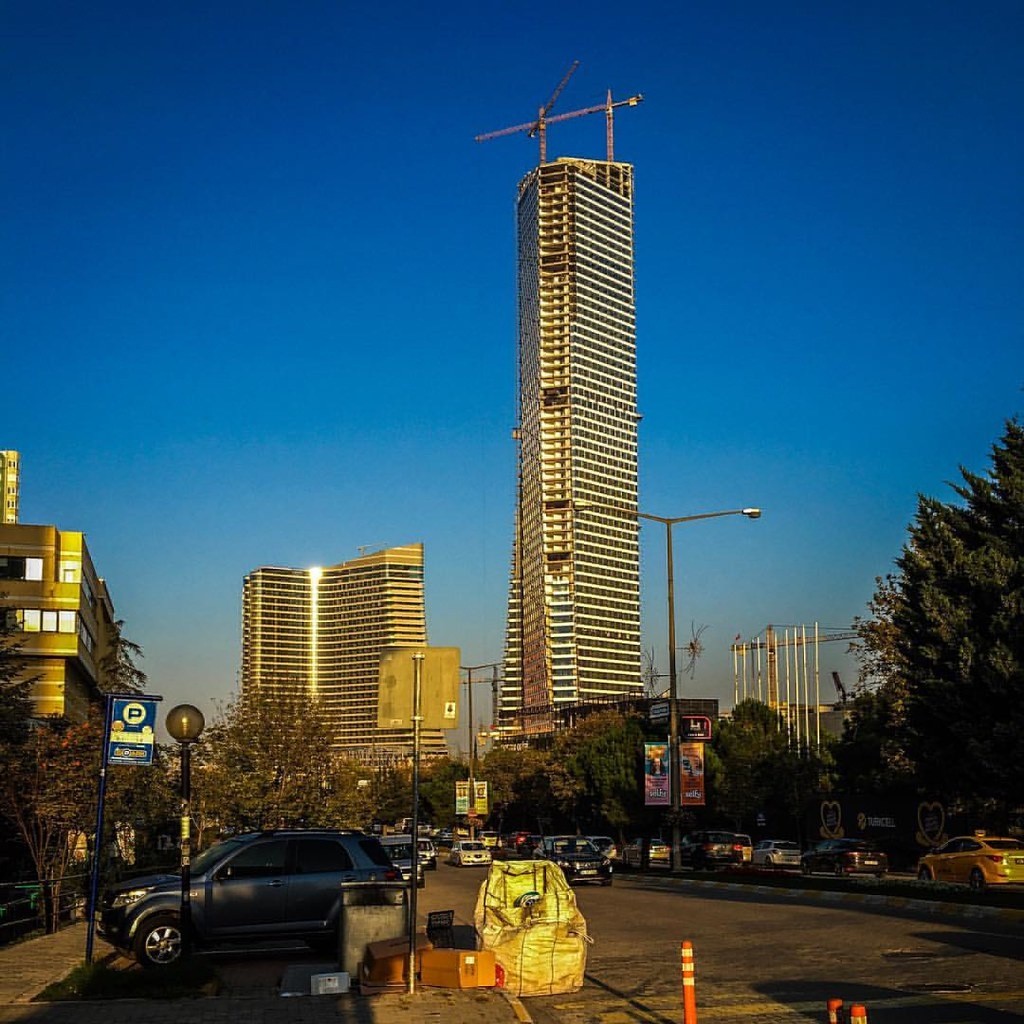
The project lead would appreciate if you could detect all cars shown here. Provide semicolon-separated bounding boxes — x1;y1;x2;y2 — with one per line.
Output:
507;830;540;858
800;838;889;877
533;836;613;887
752;838;801;869
918;830;1024;890
475;830;503;850
451;840;493;867
399;816;470;844
418;838;440;871
585;836;617;860
621;838;671;865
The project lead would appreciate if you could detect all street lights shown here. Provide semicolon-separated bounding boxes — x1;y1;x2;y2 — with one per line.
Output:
165;702;207;999
568;499;762;873
459;658;519;840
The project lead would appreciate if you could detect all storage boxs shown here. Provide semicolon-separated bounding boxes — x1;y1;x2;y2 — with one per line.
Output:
364;911;497;988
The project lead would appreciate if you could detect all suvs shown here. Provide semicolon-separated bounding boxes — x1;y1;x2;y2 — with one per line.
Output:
95;830;408;982
680;830;744;874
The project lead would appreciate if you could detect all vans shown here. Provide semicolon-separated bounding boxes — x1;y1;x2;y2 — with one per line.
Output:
379;835;429;889
733;833;754;870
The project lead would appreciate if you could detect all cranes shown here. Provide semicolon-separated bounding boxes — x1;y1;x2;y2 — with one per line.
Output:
467;52;649;167
727;622;866;711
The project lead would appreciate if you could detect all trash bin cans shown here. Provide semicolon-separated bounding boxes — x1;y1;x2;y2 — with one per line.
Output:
340;882;411;979
